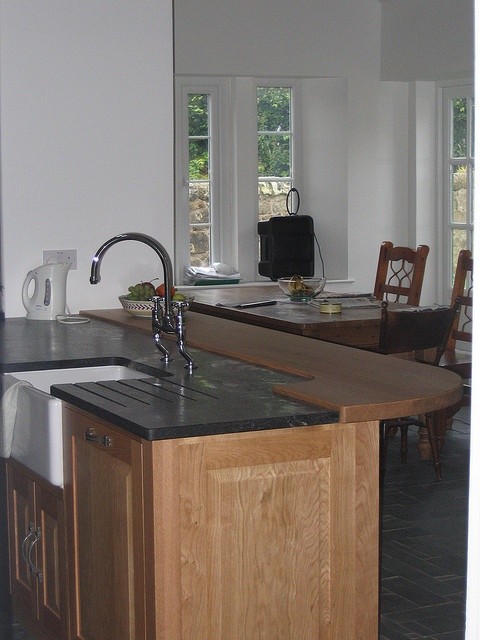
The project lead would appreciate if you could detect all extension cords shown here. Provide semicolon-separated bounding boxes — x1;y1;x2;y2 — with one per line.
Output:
22;260;73;321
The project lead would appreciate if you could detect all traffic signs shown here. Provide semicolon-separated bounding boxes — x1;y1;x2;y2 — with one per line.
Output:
43;249;77;270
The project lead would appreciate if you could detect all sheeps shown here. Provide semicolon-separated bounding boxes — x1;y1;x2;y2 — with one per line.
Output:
89;231;175;334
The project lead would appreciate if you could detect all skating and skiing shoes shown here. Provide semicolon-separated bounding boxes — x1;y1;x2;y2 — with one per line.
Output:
127;284;154;301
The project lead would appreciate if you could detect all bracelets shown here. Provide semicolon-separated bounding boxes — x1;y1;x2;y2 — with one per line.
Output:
278;277;326;302
118;296;195;318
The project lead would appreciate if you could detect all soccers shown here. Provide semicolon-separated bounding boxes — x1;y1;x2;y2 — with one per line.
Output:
156;282;175;297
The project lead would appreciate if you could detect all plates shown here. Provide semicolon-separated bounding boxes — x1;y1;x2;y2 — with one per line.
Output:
64;400;379;640
0;455;66;639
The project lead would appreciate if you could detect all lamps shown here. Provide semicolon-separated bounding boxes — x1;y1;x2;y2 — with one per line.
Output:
373;242;429;306
437;250;472;454
377;301;462;481
